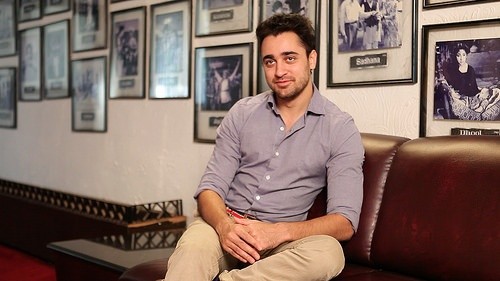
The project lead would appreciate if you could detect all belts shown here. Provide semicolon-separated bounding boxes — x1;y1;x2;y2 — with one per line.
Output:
225;204;262;221
345;21;356;24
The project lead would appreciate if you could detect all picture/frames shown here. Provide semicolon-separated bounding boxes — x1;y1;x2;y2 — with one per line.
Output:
0;0;322;144
422;0;484;8
419;18;500;137
325;0;418;88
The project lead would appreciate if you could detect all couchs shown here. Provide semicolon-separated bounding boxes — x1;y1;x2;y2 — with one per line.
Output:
118;133;500;281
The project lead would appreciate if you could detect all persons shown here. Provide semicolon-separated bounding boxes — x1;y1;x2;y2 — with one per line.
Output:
206;57;243;111
340;0;399;49
448;45;493;112
162;14;365;281
271;0;293;14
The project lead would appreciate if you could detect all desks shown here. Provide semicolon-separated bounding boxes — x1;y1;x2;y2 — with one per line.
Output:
47;228;184;281
